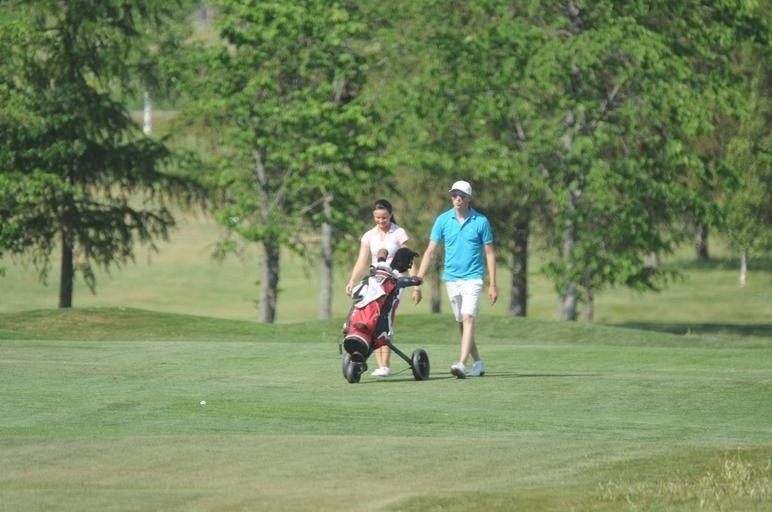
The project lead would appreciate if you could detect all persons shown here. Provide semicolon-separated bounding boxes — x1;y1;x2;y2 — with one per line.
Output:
345;199;416;379
413;180;497;378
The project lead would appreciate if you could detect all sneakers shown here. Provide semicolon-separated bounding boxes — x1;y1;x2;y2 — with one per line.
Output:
468;361;486;376
451;363;466;379
372;368;390;377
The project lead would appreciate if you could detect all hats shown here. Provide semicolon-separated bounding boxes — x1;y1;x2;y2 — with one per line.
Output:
448;180;473;197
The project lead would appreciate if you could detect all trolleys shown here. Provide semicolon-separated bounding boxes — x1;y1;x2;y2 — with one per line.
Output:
341;274;431;385
